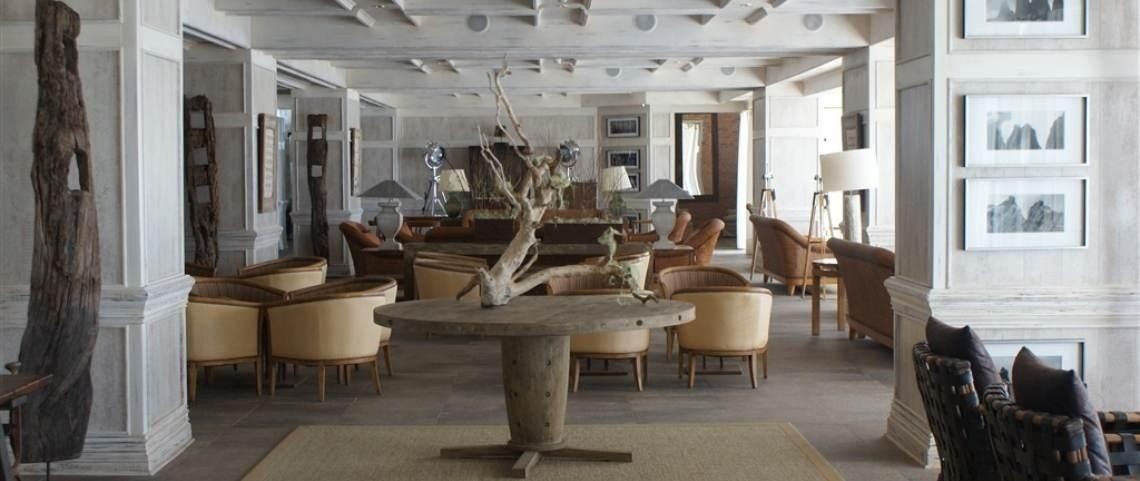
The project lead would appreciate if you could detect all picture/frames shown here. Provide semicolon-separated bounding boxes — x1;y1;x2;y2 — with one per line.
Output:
607;150;640;170
963;178;1087;251
607;117;640;138
961;1;1088;41
351;127;361;196
258;113;278;213
962;95;1090;168
628;172;640;192
841;113;863;150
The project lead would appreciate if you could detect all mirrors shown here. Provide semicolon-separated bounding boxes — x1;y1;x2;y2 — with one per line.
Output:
675;112;719;204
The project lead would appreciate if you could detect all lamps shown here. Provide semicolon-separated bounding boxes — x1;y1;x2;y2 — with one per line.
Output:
599;166;632;218
635;179;695;250
820;147;881;246
438;169;471;225
357;180;423;251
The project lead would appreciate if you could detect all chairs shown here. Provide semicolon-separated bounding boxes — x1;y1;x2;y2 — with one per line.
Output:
911;313;1140;481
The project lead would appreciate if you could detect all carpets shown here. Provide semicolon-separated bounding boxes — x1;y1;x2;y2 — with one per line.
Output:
239;421;841;481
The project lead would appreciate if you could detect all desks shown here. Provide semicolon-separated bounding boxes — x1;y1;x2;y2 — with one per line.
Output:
372;294;696;480
0;372;55;481
